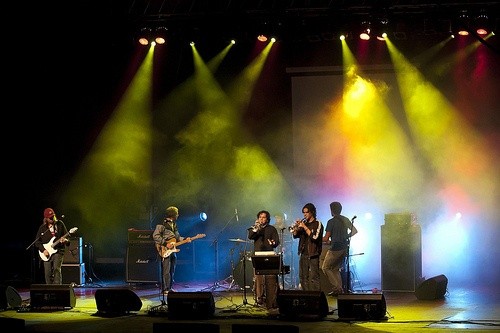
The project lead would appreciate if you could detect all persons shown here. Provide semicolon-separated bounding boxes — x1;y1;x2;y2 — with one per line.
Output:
36;208;70;284
293;203;324;290
153;206;191;293
272;211;292;265
248;210;280;305
322;202;358;296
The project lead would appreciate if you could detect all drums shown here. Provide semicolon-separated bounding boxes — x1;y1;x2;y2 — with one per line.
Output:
233;257;255;286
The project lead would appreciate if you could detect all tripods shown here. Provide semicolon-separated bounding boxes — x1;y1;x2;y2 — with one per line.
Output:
142;223;169;313
200;213;235;292
233;226;264;311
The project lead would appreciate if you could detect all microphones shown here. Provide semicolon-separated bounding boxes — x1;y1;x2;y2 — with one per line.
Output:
235;208;239;222
352;215;357;220
164;219;173;222
56;215;65;221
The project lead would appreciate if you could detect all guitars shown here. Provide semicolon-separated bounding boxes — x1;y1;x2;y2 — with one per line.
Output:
155;234;205;259
38;227;77;261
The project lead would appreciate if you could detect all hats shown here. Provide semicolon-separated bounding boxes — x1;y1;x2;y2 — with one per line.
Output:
44;208;55;218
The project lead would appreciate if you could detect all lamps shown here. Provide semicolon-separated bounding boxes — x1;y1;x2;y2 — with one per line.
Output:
139;8;491;45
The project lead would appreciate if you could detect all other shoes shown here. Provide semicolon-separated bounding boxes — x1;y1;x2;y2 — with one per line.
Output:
255;302;264;307
160;289;175;295
328;291;339;295
266;307;279;311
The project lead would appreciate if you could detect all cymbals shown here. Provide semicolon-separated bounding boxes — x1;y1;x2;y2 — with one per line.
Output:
229;239;245;242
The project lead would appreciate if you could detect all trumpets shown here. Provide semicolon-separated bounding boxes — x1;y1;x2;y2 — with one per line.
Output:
288;216;307;233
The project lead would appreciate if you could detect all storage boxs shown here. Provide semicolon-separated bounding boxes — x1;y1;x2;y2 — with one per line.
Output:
126;244;163;286
61;262;85;285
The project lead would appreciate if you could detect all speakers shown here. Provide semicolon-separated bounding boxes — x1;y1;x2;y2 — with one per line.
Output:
0;285;22;307
167;291;215;317
337;293;386;320
125;245;161;283
414;274;448;299
62;237;83;264
94;288;141;314
30;284;76;310
277;289;328;319
